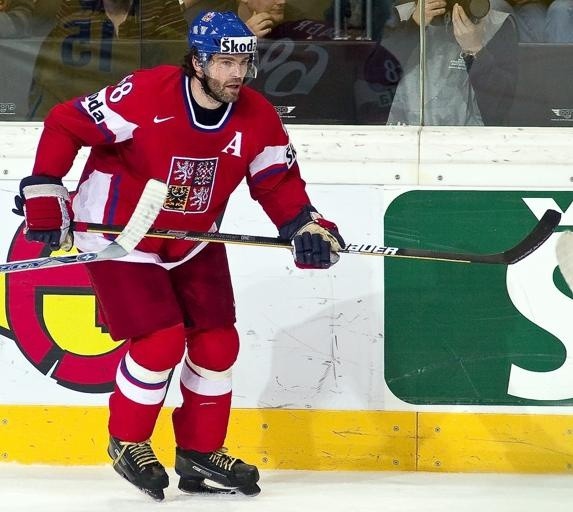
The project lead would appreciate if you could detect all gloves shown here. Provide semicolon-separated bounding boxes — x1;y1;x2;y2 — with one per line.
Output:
278;204;346;270
12;174;76;253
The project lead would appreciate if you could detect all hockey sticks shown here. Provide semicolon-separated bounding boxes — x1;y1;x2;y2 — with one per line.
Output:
0;178;167;273
69;209;561;264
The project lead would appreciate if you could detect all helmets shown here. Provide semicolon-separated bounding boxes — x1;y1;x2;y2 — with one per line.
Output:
188;8;260;80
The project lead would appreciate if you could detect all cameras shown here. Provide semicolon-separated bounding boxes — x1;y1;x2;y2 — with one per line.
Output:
428;0;490;27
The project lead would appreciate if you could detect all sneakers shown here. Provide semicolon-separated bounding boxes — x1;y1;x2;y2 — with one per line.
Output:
175;446;259;487
108;440;170;490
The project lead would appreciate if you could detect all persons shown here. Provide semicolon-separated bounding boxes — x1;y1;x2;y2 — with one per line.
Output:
1;1;573;128
17;7;346;492
